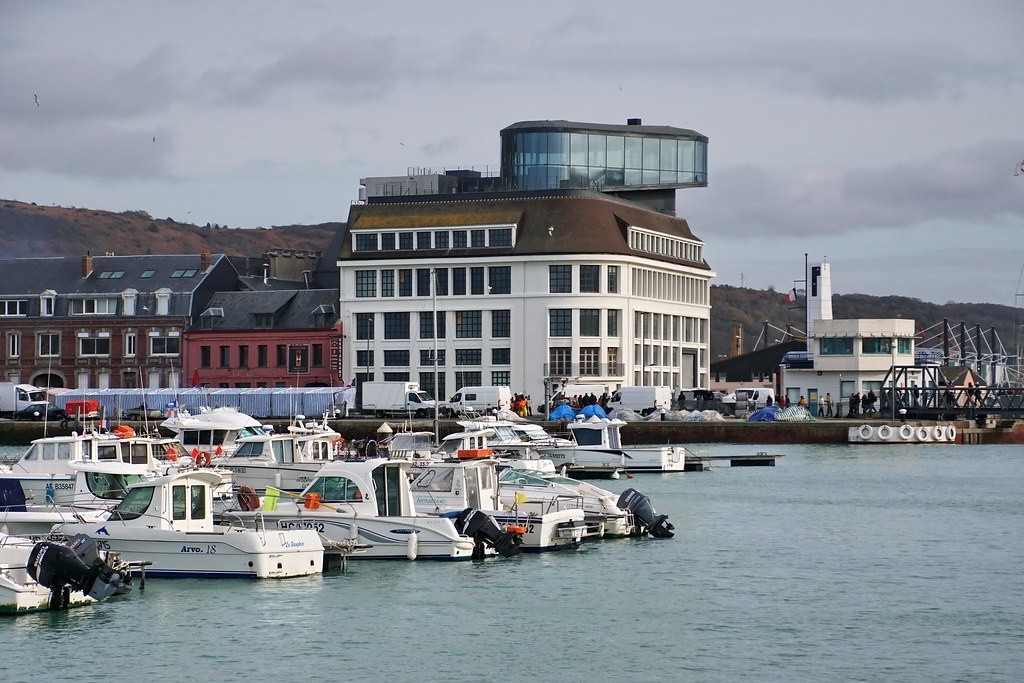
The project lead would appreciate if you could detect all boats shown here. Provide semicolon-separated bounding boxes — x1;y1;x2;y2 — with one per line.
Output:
0;321;688;580
0;504;153;617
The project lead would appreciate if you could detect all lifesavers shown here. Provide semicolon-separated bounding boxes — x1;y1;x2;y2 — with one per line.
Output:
332;437;346;451
237;484;260;511
899;425;914;439
946;426;956;440
931;426;944;440
196;452;211;466
166;448;178;461
507;526;525;533
916;427;930;441
859;425;873;440
878;425;892;439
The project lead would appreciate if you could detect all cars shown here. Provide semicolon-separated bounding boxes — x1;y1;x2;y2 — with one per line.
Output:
12;403;66;422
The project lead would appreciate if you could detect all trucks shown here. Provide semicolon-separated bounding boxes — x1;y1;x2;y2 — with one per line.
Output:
0;380;48;420
362;381;436;419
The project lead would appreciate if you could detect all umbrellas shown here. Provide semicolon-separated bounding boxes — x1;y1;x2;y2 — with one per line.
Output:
192;367;200;388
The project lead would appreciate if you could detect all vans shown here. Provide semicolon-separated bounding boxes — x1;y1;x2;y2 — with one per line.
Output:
444;385;512;419
603;386;672;421
722;387;776;414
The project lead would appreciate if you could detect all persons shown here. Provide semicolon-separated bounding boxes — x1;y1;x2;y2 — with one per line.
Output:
766;394;791;409
819;393;834;418
797;395;807;408
849;384;928;415
944;380;985;407
678;391;685;411
510;393;533;417
573;392;609;411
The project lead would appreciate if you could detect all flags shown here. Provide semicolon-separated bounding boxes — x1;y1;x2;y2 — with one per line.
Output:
215;445;222;455
98;420;110;429
786;288;797;304
191;448;199;459
263;486;279;511
